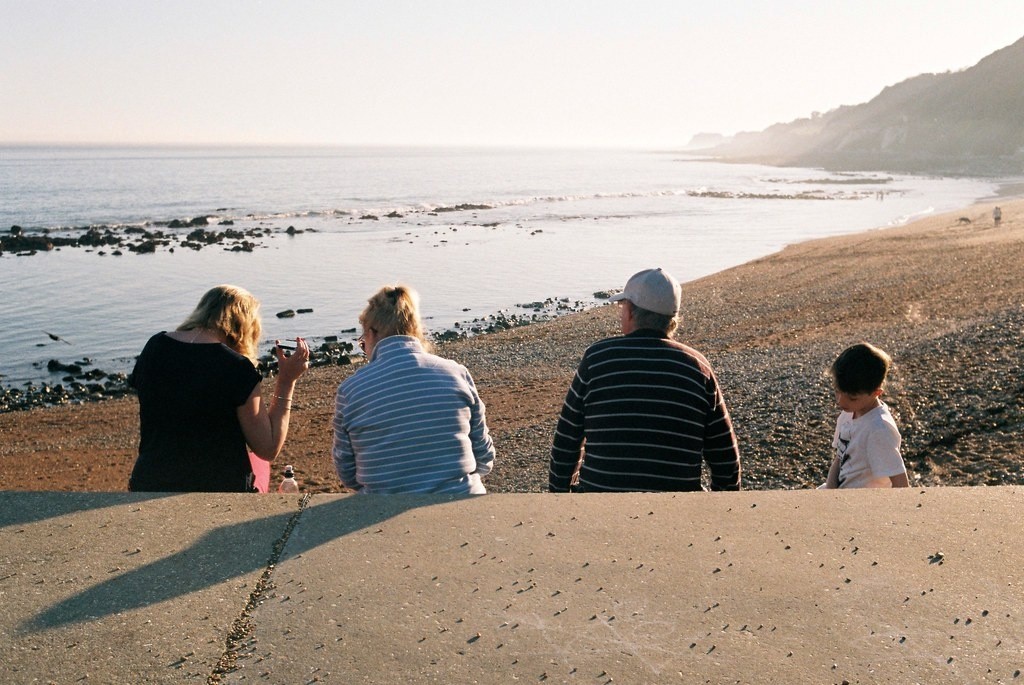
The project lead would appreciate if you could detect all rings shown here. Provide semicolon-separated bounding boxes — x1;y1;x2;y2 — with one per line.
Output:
303;357;309;362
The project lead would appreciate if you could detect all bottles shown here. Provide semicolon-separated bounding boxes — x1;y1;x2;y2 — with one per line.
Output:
279;465;299;493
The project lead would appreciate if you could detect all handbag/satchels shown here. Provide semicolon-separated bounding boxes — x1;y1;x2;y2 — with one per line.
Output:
248;452;270;493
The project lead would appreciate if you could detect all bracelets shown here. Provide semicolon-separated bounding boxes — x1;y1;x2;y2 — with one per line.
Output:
270;393;292;401
270;402;291;411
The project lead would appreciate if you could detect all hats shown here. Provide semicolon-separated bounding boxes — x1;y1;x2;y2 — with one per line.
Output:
609;268;682;315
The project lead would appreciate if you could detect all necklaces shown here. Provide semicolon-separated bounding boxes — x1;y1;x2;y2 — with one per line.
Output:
190;326;217;343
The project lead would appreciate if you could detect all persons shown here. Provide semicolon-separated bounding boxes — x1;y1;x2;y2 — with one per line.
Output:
331;284;496;494
549;267;741;493
816;342;909;488
992;207;1003;227
128;284;310;494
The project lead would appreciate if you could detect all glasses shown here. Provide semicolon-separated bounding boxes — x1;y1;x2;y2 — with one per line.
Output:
357;327;377;352
617;300;632;309
